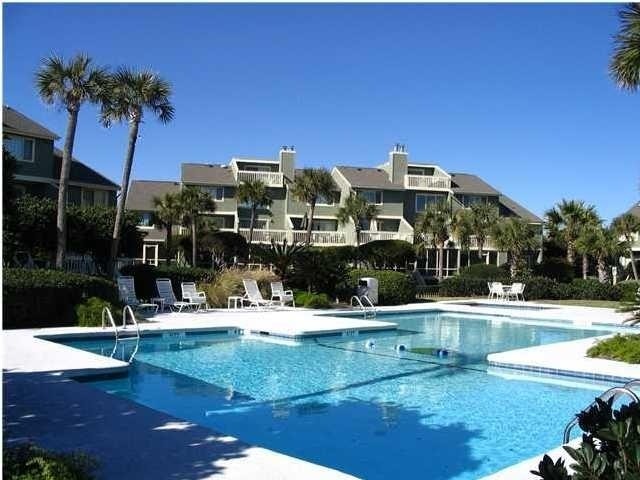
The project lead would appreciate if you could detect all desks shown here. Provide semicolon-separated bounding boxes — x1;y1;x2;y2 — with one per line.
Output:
150;298;165;312
228;296;243;309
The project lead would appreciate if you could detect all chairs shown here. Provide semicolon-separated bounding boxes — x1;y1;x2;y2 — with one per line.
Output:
118;276;159;314
270;281;295;307
242;279;280;307
487;281;526;302
155;278;201;314
181;282;207;311
